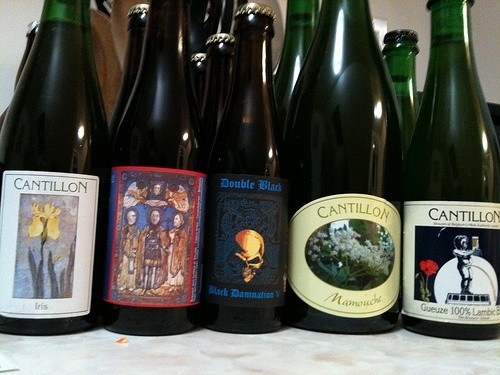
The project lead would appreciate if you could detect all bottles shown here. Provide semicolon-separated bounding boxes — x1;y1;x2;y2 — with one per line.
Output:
402;0;500;340
1;0;109;335
201;4;288;335
272;0;320;143
103;0;209;337
383;31;420;156
15;19;85;104
107;4;151;146
205;34;235;143
189;52;207;108
285;0;405;334
